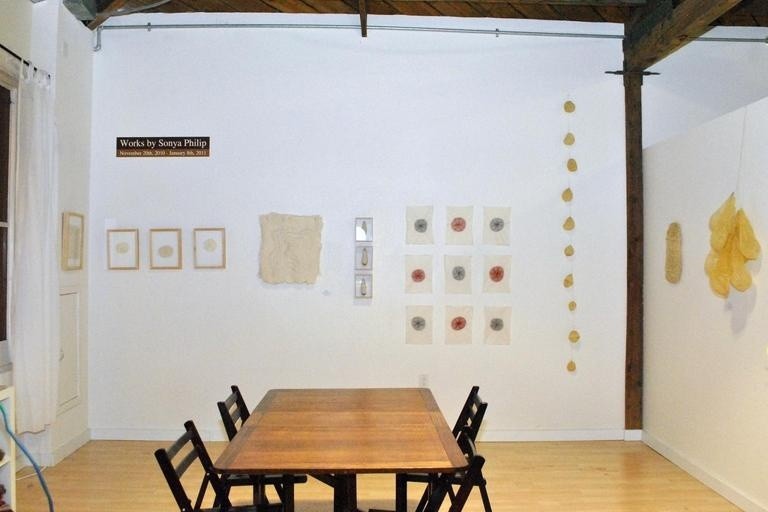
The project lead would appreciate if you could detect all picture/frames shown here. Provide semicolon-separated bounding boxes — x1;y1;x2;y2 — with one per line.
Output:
355;217;374;299
61;210;226;272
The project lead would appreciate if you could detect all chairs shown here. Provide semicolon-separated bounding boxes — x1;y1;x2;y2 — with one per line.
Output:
395;384;488;512
368;427;493;511
153;419;310;511
216;385;297;510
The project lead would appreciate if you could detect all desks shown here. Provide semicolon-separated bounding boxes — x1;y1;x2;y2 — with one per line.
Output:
212;387;468;510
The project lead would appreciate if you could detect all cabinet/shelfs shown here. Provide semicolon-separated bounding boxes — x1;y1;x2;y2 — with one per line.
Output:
0;385;17;512
